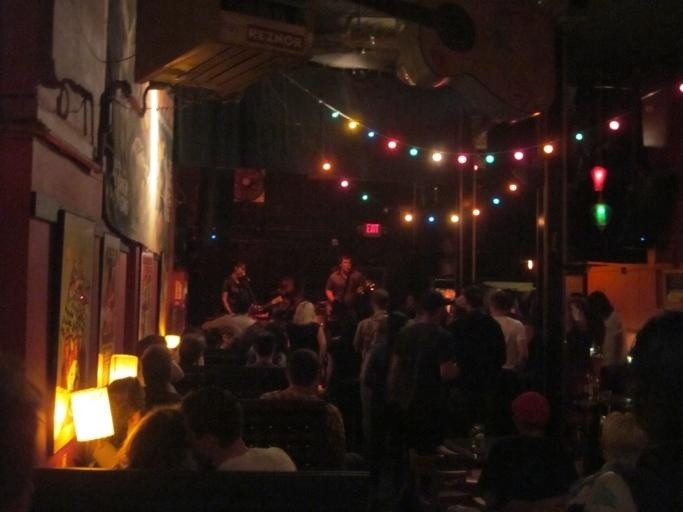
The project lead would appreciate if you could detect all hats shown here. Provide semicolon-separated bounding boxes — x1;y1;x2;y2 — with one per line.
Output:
603;410;646;448
512;391;551;424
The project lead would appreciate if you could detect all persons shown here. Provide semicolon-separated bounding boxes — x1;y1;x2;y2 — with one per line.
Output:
74;254;683;512
0;352;49;512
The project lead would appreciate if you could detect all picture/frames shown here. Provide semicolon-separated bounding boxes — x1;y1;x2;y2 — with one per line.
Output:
46;208;124;460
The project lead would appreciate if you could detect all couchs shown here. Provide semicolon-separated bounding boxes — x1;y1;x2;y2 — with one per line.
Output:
28;364;386;510
